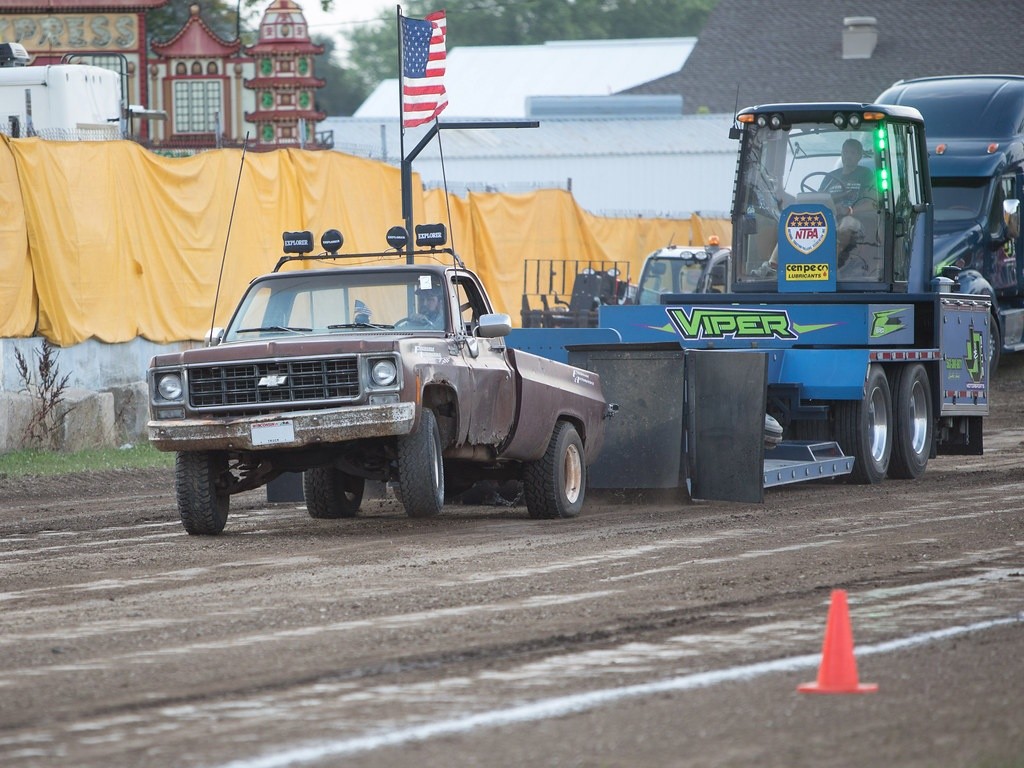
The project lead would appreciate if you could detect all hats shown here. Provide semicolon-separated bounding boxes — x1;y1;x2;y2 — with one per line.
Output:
415;284;441;296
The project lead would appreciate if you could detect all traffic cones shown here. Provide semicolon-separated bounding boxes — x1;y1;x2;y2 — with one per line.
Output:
799;588;879;694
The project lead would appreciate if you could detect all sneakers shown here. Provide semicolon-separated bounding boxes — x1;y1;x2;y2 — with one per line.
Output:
750;263;776;280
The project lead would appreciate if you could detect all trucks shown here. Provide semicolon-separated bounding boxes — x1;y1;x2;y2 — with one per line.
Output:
869;73;1024;380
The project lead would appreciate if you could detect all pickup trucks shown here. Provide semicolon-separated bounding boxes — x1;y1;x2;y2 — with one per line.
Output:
143;223;607;529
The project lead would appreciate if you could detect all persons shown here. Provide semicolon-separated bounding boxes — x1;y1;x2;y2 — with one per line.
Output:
748;139;877;278
402;281;446;330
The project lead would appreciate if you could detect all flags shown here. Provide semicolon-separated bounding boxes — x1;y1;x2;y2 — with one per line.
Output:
399;9;449;129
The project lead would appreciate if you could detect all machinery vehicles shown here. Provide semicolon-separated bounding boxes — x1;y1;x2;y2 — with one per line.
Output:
505;98;988;485
